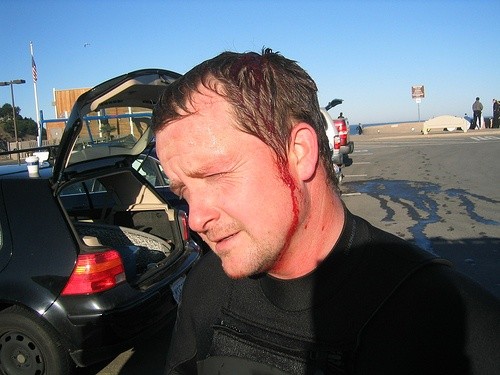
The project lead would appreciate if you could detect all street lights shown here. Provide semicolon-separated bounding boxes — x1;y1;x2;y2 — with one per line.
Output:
0;79;26;165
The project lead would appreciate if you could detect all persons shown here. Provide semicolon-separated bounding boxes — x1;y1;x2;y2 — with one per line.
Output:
493;99;497;126
474;98;482;130
463;112;493;129
337;112;345;119
496;100;499;128
153;47;500;375
358;122;363;135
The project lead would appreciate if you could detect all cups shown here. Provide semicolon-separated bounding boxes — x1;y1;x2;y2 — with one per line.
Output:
25;156;39;177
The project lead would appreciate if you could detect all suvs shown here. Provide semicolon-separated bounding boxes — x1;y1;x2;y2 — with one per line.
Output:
316;106;355;185
0;67;211;375
